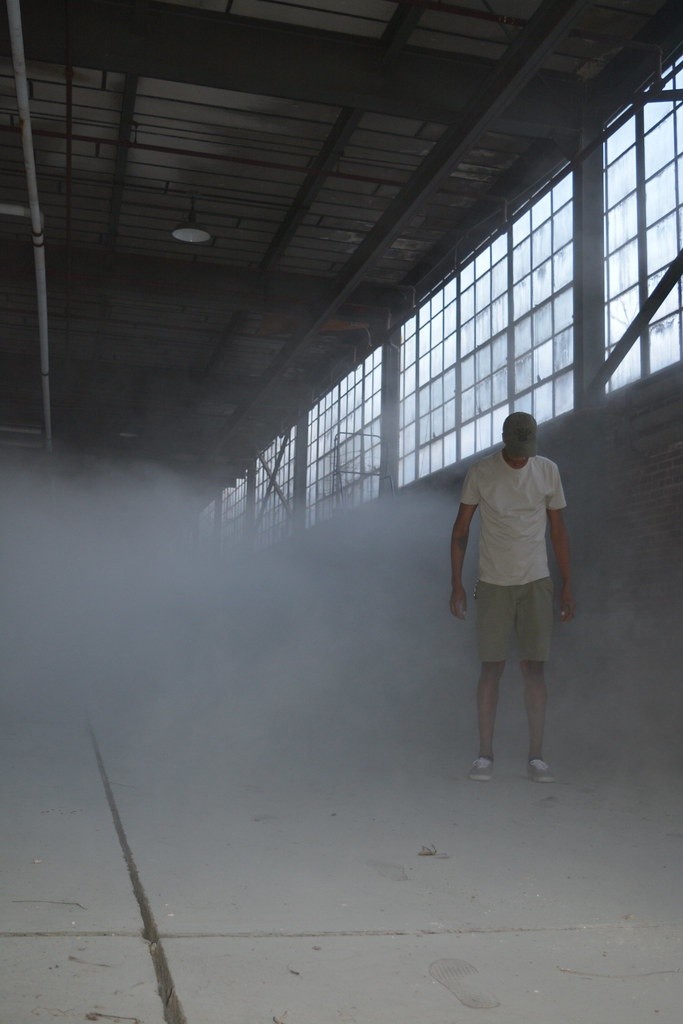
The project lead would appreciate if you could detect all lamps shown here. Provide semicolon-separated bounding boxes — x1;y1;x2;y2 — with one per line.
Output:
169;197;213;243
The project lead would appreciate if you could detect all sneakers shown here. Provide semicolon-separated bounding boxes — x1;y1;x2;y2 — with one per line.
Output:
529;758;557;783
467;755;493;781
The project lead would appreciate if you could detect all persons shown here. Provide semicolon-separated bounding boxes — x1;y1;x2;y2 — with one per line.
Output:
449;412;577;783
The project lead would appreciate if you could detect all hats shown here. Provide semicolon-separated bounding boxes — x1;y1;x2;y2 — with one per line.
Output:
503;410;539;458
